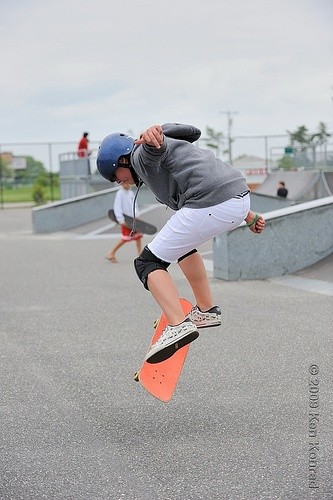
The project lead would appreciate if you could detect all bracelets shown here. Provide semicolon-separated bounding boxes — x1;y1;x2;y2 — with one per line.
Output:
246;214;259;227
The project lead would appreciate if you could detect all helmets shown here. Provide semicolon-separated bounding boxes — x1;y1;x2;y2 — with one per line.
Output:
96;133;135;182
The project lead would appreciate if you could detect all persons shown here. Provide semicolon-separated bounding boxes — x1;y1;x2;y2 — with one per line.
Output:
97;123;265;364
105;183;143;264
277;182;288;198
78;133;89;158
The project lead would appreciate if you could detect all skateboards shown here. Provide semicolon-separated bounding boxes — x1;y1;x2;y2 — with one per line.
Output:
108;209;157;234
133;298;194;402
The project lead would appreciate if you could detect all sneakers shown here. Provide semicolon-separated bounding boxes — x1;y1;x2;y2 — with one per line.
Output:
185;305;222;328
146;316;200;364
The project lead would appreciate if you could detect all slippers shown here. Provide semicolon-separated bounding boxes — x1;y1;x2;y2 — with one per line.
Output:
105;254;117;263
